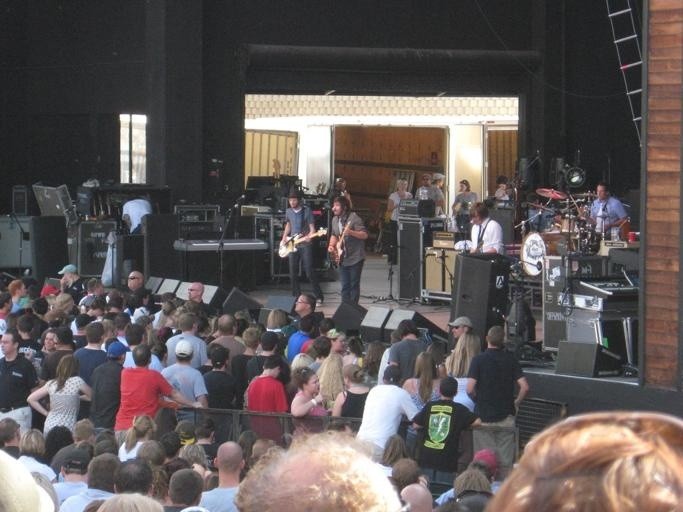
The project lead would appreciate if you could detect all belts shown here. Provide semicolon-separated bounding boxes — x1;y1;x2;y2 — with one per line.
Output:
0;403;28;412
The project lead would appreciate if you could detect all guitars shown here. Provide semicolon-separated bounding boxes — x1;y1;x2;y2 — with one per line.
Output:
278;227;327;258
328;220;352;266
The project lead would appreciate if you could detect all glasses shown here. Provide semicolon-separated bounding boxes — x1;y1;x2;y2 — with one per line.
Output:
455;489;492;501
129;276;138;280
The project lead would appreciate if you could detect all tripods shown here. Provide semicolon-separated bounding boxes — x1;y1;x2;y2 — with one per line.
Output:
372;243;404;306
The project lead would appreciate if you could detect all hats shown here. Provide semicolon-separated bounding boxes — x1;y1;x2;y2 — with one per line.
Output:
212;347;228;362
75;314;96;327
57;264;79;274
448;315;473;328
175;420;195;445
107;342;130;355
488;326;504;344
136;315;155;326
175;340;192;357
64;450;89;469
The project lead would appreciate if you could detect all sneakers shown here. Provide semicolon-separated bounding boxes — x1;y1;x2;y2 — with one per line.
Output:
316;299;322;307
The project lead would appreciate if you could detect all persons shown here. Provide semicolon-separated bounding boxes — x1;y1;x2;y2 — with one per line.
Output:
387;178;414;266
0;265;683;511
121;196;155;264
327;195;369;305
415;174;434;201
468;202;504;256
428;172;448;217
452;179;478;232
588;181;629;241
335;178;352;209
281;192;324;308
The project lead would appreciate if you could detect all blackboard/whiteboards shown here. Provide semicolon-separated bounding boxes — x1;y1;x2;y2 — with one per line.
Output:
78;221;117;278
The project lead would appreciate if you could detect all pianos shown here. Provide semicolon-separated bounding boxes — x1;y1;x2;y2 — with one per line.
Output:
174;237;269;252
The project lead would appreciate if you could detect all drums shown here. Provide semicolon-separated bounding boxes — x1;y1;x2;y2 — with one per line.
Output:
520;230;565;276
562;214;597;232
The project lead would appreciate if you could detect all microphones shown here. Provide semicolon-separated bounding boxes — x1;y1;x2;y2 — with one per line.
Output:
229;205;238;211
233;193;246;205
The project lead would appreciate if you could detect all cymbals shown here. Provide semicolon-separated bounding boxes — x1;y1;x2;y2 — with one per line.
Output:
536;188;567;200
527;201;556;214
572;193;596;198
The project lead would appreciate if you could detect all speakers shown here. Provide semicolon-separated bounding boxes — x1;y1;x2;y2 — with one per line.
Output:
555;341;624;379
331;299;369;337
222;286;264;323
258;295;297;329
145;276;224;310
360;306;393;342
446;251;510;355
28;214;69;279
383;308;449;354
